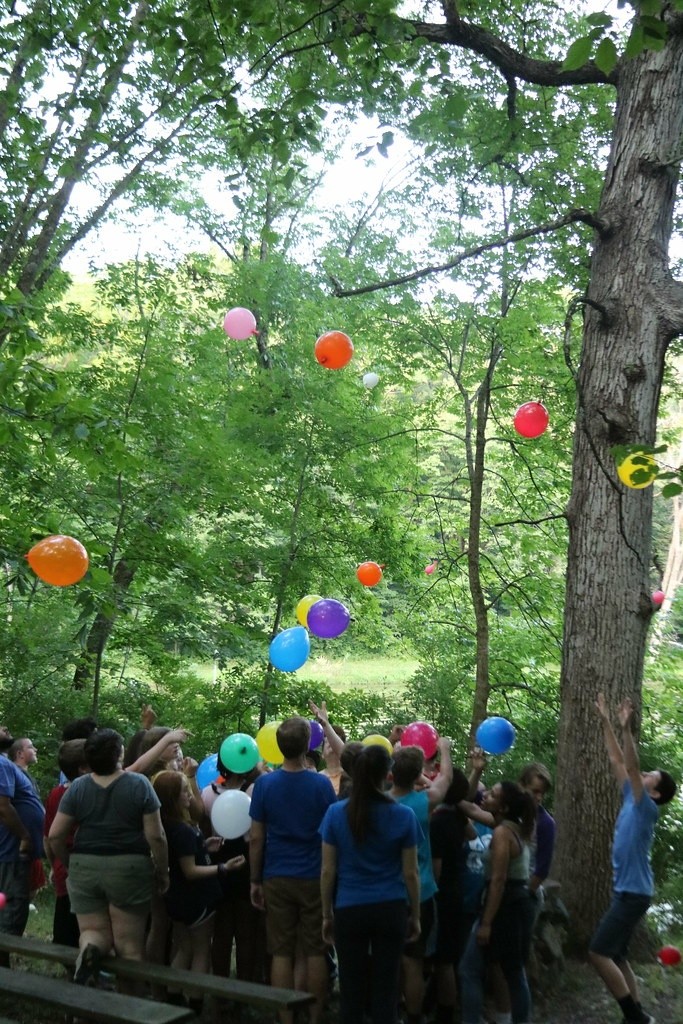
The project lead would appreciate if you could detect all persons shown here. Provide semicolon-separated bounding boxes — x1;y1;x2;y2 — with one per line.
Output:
588;691;677;1024
0;699;556;1024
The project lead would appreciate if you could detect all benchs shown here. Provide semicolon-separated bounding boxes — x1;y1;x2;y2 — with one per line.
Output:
0;932;316;1024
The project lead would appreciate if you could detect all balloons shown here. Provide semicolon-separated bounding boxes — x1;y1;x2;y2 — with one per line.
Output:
401;721;439;761
361;734;393;757
362;373;379;388
296;594;324;627
307;599;351;638
313;329;354;369
210;790;252;841
652;591;665;604
25;535;89;587
617;451;656;489
476;717;516;756
195;721;324;790
268;626;310;672
658;945;682;966
357;561;383;586
224;308;259;340
514;399;549;438
425;566;434;574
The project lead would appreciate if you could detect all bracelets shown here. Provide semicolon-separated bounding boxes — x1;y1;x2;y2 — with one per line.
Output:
218;863;221;875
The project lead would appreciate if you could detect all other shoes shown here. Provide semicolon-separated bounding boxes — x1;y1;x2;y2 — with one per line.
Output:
76;944;102;988
621;1012;655;1024
